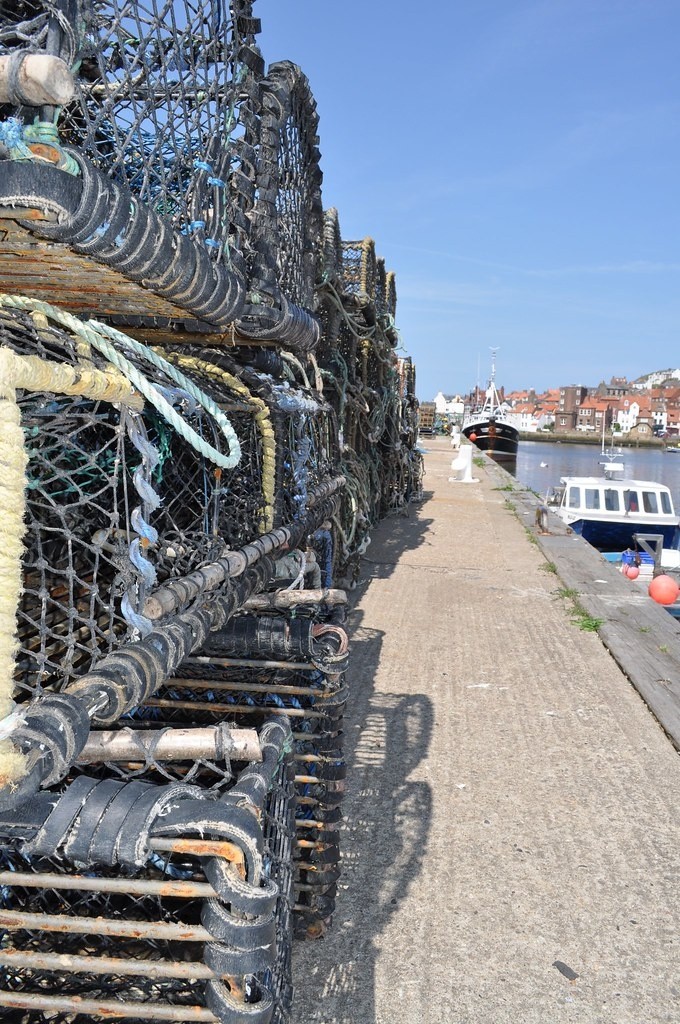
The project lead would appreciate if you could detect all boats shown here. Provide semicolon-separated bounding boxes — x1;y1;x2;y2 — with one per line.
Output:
548;475;680;615
461;346;519;463
